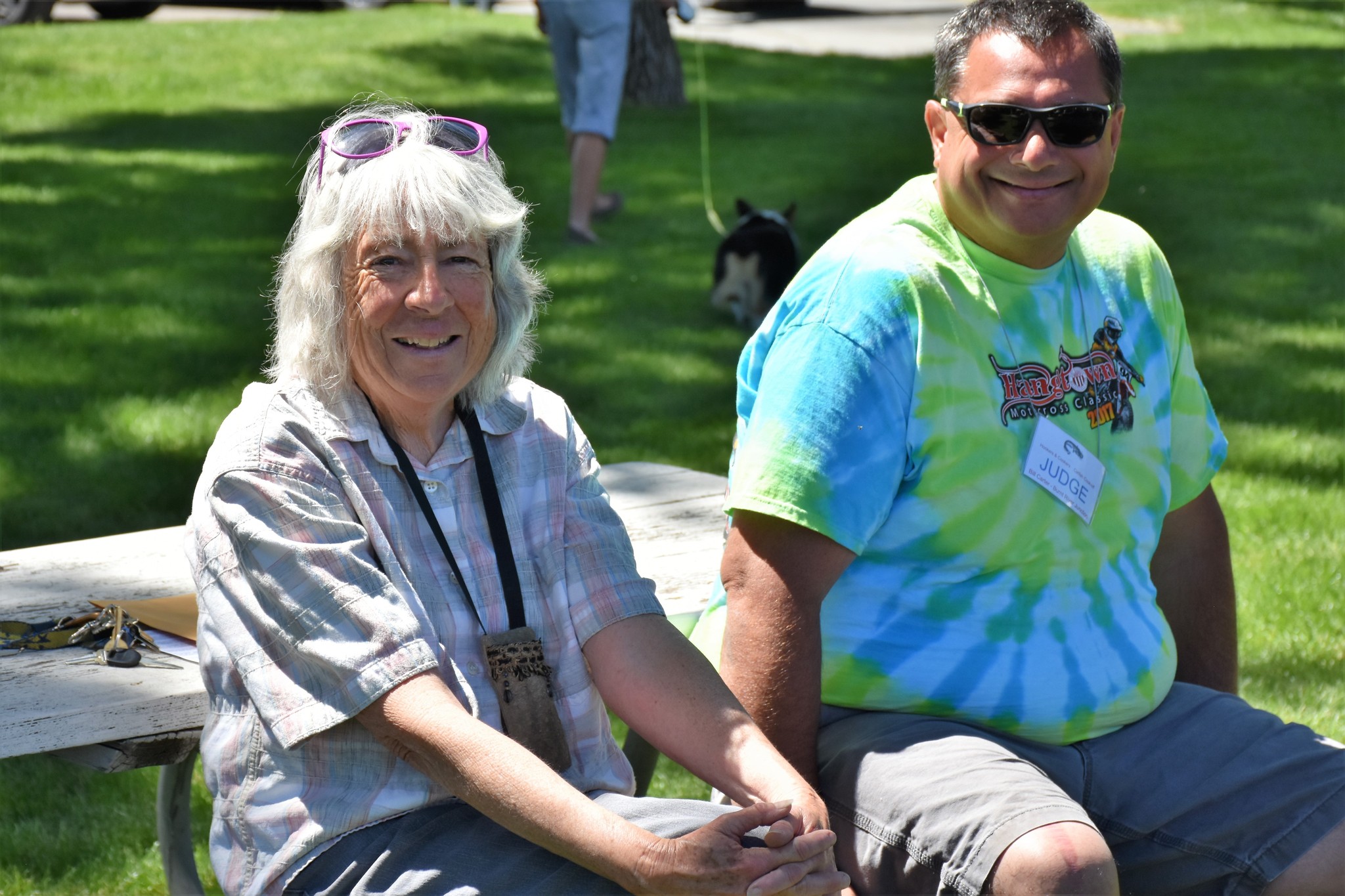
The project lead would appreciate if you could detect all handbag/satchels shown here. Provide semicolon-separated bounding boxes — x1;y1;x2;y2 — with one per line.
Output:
479;625;575;776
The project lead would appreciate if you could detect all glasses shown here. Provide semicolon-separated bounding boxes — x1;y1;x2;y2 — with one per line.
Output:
934;94;1116;148
315;116;490;191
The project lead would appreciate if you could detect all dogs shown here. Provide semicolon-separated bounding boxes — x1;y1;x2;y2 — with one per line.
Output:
710;197;802;327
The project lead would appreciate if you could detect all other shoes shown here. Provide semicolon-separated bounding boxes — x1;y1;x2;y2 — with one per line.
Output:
591;189;622;216
566;222;603;246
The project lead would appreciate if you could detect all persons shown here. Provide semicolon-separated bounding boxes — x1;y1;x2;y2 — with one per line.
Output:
723;1;1344;896
183;99;852;895
536;0;635;242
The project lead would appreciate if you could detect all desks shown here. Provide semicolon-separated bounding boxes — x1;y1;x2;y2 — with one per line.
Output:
1;461;737;895
